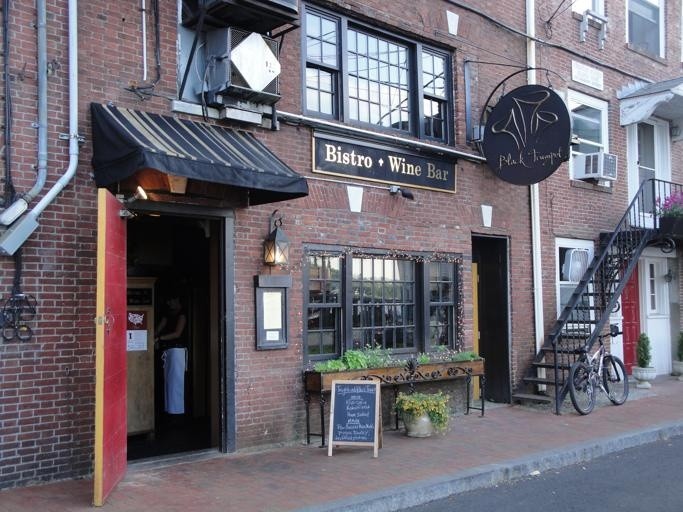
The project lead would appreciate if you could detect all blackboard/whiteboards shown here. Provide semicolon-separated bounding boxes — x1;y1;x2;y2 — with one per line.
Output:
329;380;379;446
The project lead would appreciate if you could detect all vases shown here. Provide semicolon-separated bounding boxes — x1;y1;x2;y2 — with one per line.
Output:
402;410;438;440
659;213;682;233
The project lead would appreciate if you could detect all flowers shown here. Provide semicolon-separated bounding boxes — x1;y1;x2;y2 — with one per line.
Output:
649;185;683;216
390;387;455;436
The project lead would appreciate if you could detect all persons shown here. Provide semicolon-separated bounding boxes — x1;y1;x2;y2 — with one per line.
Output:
154;292;191;424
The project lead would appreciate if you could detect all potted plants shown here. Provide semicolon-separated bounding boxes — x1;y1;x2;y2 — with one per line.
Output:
670;330;683;376
630;334;656;390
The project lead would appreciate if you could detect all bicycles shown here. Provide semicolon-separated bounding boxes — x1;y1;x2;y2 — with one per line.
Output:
565;328;629;414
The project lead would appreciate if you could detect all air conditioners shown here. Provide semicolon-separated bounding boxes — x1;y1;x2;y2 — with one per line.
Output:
573;153;620;182
203;28;280;98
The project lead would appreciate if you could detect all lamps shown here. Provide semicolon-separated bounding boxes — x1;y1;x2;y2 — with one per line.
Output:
662;269;676;282
115;185;150;204
386;186;417;203
261;207;292;278
